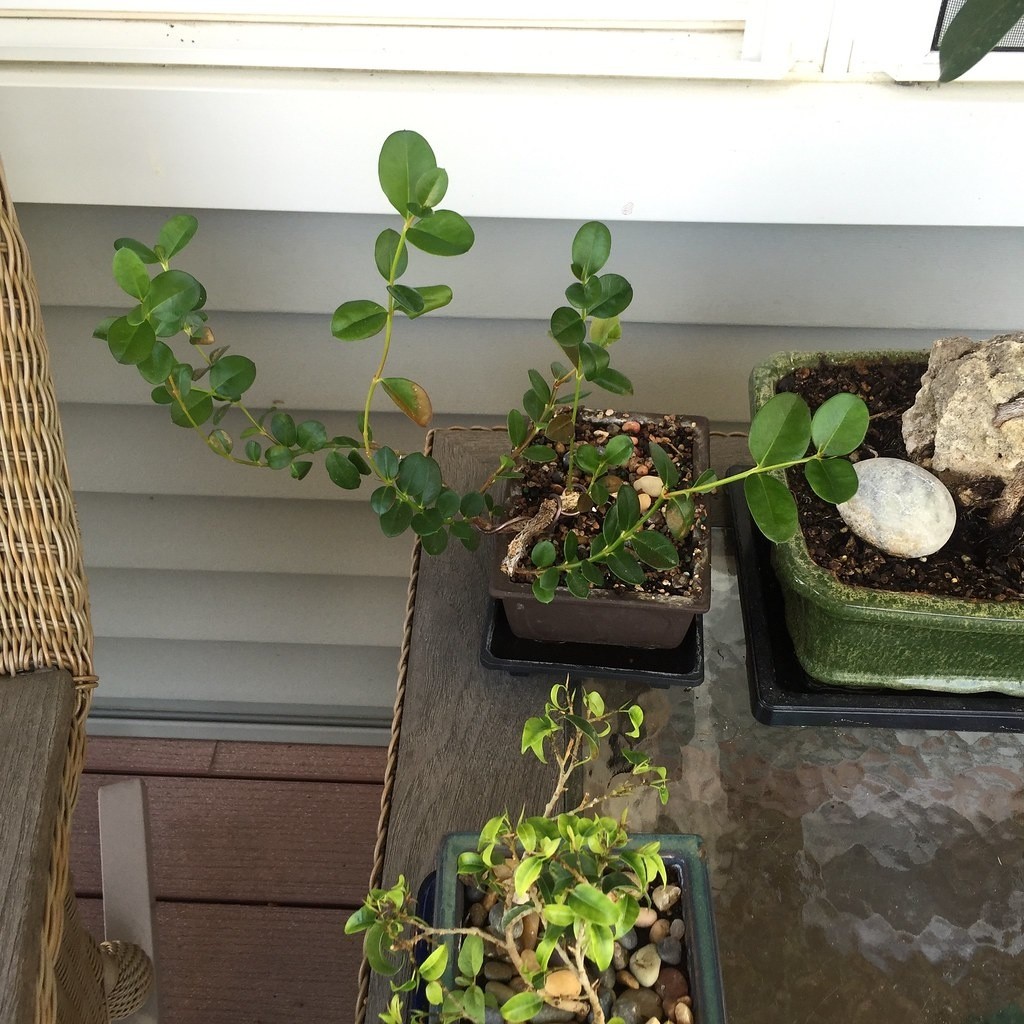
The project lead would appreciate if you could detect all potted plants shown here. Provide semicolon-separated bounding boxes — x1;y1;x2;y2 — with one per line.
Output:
344;678;724;1024
91;136;864;649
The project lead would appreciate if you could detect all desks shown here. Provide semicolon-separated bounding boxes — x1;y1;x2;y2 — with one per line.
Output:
351;415;1024;1024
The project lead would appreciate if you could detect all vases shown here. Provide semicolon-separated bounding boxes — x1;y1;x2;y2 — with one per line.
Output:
748;330;1024;699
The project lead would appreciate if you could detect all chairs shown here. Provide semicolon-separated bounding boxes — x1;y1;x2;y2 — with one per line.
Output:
0;174;160;1024
